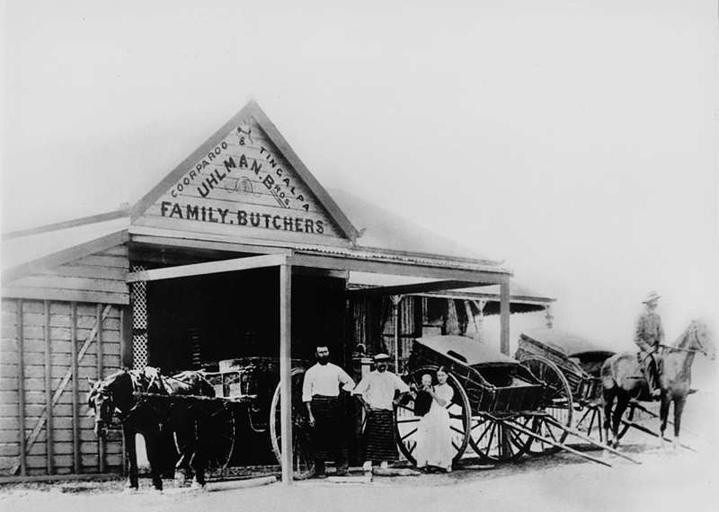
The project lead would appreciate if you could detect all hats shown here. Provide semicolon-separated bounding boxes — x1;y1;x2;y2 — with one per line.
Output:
373;353;393;362
642;289;664;304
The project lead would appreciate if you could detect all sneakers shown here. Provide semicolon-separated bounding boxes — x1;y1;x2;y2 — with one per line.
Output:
305;461;391;478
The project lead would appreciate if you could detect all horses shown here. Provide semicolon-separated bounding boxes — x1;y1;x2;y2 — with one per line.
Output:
599;318;716;450
86;363;216;494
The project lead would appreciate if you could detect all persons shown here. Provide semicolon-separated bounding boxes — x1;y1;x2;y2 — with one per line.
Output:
632;290;670;399
413;363;456;473
414;372;435;416
301;343;357;480
352;349;411;477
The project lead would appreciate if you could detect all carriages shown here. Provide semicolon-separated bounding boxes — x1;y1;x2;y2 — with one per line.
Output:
88;355;323;490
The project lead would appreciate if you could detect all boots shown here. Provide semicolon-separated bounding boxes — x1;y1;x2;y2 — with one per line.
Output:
647;372;664;398
